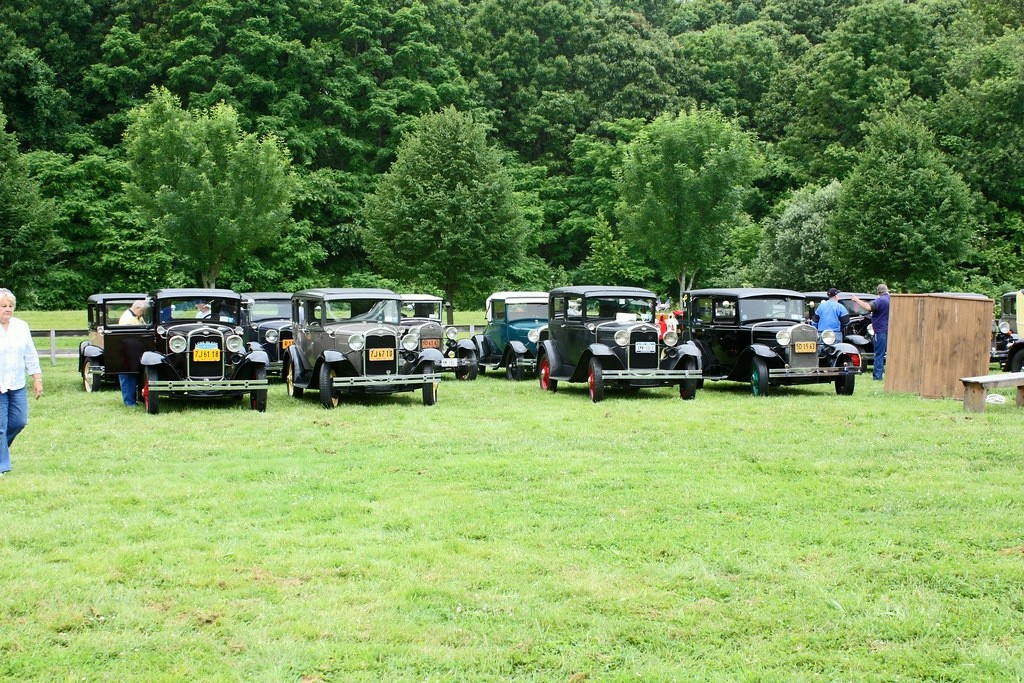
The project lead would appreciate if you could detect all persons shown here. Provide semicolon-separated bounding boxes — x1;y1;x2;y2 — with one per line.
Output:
849;283;890;380
118;300;149;407
0;288;43;478
811;288;850;367
195;300;212;319
566;297;583;318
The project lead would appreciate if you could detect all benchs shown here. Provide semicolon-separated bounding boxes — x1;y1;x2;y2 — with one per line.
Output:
959;371;1024;412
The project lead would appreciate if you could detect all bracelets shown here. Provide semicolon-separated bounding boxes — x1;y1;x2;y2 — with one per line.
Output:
34;379;42;382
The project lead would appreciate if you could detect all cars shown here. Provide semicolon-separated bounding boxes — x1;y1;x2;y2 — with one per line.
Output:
991;291;1024;374
75;283;888;415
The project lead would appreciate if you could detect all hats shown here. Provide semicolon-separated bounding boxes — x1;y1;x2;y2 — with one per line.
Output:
827;288;840;297
